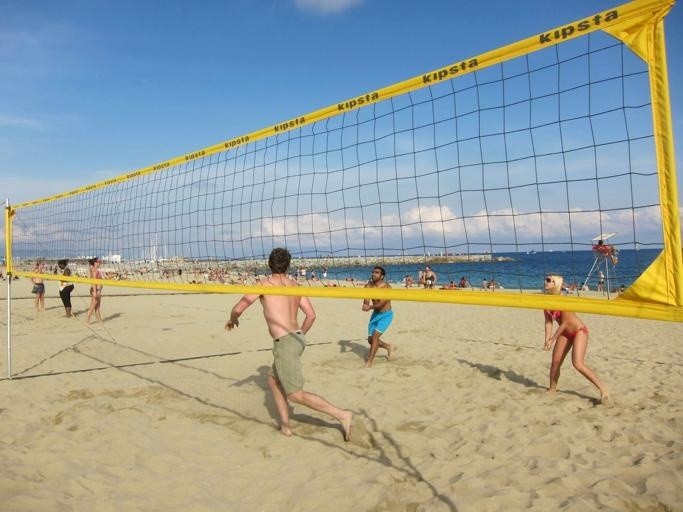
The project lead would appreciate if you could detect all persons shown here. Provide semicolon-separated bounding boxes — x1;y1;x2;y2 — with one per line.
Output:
31;261;46;310
224;246;352;442
541;273;610;403
1;239;507;293
592;240;618;265
84;257;105;324
360;266;396;367
57;258;75;317
562;281;626;293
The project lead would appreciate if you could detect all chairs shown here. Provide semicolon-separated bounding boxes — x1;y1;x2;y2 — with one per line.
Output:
591;244;619;264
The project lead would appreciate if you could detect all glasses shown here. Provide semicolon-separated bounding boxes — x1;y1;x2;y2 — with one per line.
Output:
546;278;554;282
